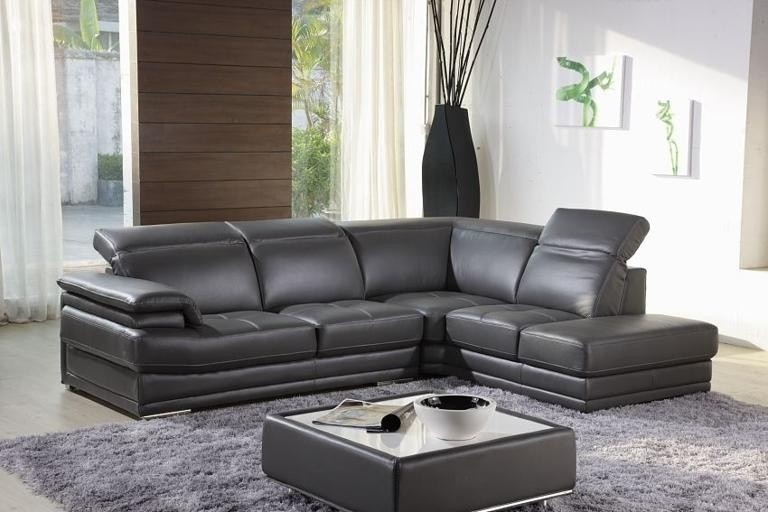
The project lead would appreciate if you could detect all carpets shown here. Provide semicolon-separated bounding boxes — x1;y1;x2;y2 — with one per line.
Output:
0;370;768;512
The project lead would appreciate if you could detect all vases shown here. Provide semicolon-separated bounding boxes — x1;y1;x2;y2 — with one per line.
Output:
418;104;481;220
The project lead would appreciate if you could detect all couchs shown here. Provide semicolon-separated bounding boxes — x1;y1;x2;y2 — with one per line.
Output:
53;206;720;421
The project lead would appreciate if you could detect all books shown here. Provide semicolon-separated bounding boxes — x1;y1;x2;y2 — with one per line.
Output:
313;397;406;429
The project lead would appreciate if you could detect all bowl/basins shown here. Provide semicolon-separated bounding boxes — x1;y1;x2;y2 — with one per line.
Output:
412;394;496;441
